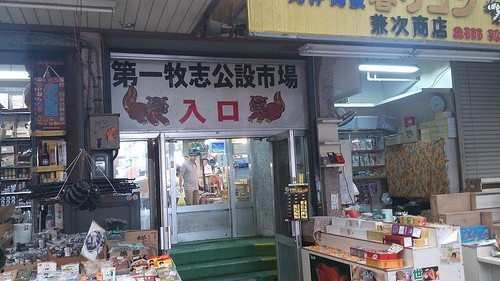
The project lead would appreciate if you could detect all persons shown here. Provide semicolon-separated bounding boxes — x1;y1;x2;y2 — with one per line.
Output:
136;171;149;210
176;149;224;206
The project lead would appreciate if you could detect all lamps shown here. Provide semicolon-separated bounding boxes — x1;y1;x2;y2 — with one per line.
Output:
415;49;500;62
298;42;413;60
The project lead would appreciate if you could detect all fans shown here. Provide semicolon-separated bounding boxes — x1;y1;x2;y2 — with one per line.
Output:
335;107;356;127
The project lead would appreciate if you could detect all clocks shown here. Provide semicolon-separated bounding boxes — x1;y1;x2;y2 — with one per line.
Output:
430;95;445;114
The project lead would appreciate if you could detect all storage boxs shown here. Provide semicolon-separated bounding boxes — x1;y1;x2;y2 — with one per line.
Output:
107;230;159;258
327;215;430;269
48;238;107;266
339;115;379;131
385;125;420;149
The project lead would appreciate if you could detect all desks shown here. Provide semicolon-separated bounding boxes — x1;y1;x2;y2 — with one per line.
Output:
0;252;183;281
41;190;142;232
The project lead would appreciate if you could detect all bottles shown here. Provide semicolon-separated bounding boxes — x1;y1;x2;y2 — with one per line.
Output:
42;143;49;165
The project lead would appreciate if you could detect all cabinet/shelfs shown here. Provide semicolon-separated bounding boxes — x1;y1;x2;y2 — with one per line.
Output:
317;119;346;168
31;130;67;172
352;149;387;182
0;110;33;223
